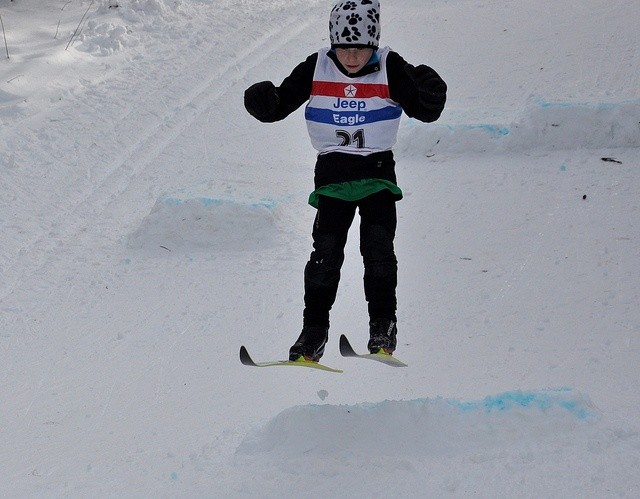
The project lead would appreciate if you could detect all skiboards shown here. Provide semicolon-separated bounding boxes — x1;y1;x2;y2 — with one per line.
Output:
240;334;407;373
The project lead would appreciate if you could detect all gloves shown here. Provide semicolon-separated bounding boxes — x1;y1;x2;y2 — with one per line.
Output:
413;64;446;111
244;81;280;121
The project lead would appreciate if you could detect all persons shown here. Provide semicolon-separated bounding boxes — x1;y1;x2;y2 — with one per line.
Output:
241;0;448;362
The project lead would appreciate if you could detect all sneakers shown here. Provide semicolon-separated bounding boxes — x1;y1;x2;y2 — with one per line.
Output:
367;322;397;357
289;317;329;362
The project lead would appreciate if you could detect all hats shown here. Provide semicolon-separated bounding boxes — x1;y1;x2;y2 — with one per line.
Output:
328;0;380;50
